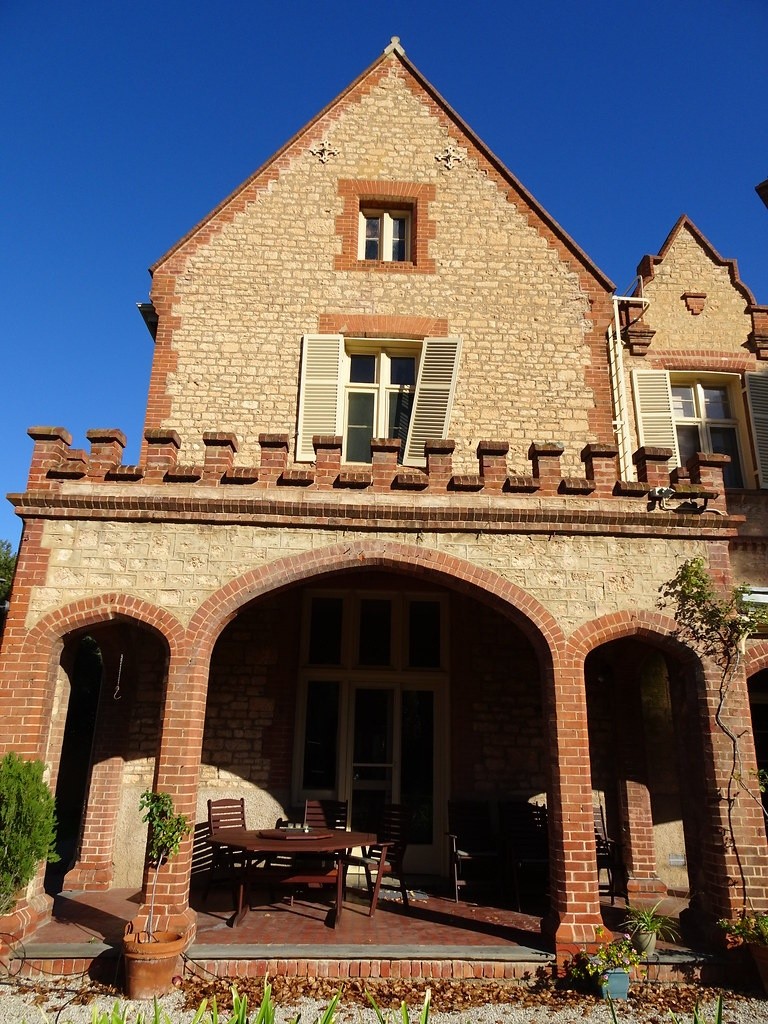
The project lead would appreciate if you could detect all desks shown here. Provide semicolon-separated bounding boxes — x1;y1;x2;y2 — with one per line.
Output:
208;829;377;928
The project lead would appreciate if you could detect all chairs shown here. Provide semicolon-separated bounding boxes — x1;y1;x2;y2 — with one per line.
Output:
203;798;409;917
445;800;621;912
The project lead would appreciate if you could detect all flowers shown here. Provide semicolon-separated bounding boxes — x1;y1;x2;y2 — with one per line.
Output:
571;924;647;985
719;910;768;948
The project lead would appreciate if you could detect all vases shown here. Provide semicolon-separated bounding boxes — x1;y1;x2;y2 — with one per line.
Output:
748;945;768;995
601;968;629;1000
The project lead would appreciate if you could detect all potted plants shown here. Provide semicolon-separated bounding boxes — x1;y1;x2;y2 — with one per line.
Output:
123;792;191;1000
619;887;694;956
659;558;768;985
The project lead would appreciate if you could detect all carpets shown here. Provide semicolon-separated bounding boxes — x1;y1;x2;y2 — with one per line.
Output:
360;882;429;899
